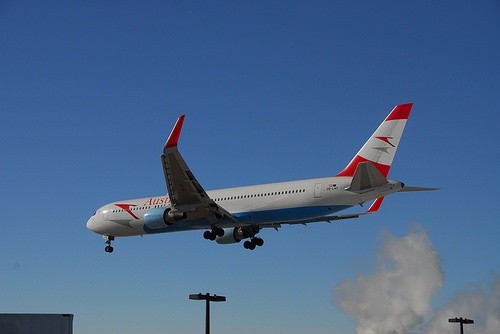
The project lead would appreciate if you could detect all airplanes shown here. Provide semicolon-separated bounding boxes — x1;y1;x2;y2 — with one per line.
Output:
85;102;441;251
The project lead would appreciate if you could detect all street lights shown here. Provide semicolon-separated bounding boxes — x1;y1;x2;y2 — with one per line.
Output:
449;317;474;334
189;292;227;334
